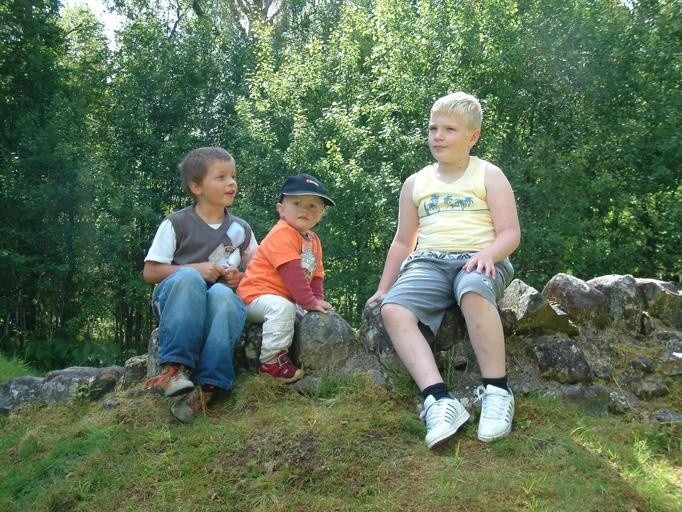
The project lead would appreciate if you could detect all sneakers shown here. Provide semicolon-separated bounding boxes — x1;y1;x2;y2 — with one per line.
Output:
145;365;195;396
473;382;515;444
259;354;301;384
418;391;471;449
171;384;220;424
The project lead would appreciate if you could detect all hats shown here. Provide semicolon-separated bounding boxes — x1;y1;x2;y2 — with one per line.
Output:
279;174;337;206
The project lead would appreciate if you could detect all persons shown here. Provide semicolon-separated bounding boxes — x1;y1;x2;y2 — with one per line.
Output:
234;174;336;382
364;91;525;451
144;146;261;427
